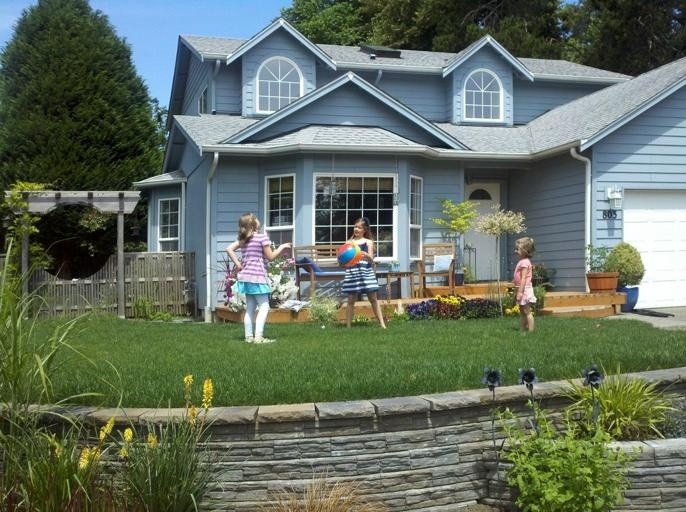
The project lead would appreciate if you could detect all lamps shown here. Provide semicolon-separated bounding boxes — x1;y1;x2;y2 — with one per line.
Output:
604;183;624;213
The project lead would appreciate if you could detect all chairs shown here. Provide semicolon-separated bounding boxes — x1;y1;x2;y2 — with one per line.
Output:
415;243;456;297
293;245;339;301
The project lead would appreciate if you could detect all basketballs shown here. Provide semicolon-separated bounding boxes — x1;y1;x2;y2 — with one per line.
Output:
337;242;362;268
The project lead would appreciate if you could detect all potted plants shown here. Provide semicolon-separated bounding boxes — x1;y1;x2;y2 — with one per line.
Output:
423;191;482;285
584;242;620;293
608;243;645;313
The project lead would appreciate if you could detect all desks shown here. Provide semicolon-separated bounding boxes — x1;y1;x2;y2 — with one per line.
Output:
314;271;416;301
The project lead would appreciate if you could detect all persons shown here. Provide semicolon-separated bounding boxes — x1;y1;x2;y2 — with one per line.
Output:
226;212;295;343
342;218;387;329
514;237;537;337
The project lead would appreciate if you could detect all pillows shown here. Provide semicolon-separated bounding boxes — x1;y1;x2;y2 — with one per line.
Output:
296;257;321;273
433;253;452;272
